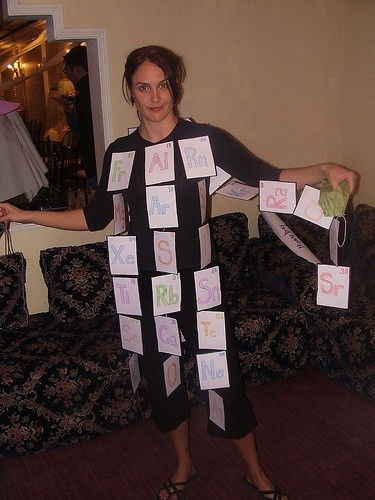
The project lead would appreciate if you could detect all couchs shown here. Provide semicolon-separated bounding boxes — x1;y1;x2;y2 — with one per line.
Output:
0;236;375;456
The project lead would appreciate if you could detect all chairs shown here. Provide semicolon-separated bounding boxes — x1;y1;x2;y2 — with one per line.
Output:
25;120;88;212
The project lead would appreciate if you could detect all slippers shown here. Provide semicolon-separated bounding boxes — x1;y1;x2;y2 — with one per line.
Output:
158;470;195;500
244;474;287;500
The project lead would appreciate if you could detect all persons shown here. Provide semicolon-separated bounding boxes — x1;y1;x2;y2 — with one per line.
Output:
58;46;98;188
1;45;361;500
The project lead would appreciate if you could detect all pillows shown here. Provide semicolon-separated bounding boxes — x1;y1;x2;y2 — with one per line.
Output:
210;212;256;289
39;240;117;323
0;252;28;334
258;202;353;264
350;203;375;272
